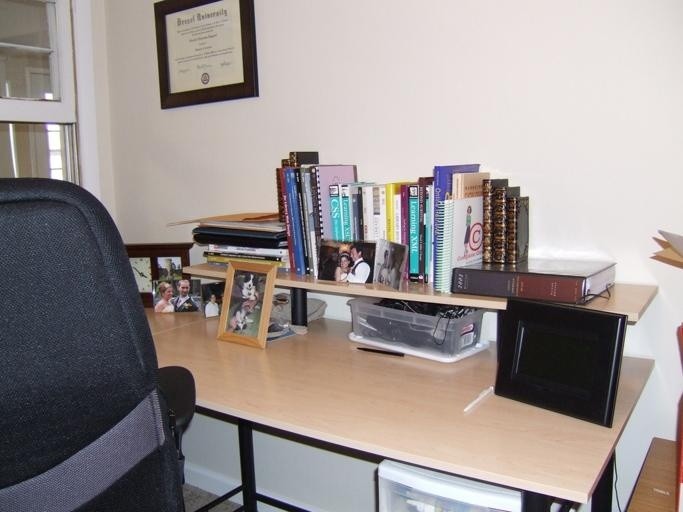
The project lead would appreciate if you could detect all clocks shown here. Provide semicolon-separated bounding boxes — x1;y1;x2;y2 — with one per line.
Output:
124;245;157;307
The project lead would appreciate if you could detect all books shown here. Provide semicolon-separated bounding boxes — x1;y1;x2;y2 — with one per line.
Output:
493;297;628;428
448;259;618;305
190;149;531;294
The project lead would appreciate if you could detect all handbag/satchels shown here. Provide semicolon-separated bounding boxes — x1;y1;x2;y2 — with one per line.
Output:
269;293;327;327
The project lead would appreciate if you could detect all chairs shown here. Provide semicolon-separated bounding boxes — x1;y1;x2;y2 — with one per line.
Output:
0;178;195;511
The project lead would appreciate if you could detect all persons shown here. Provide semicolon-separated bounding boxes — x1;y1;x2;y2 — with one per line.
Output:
155;282;173;311
169;279;197;311
203;293;219;318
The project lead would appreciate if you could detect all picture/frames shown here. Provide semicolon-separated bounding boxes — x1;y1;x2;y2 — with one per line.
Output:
152;1;259;112
215;258;280;351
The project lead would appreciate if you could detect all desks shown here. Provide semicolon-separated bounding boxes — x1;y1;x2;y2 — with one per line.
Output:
137;263;658;512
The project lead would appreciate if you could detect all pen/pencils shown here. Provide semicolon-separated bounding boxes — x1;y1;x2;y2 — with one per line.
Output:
356;346;405;357
